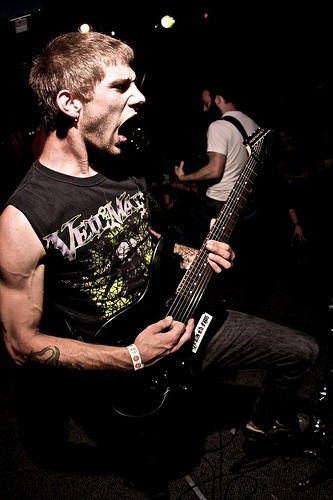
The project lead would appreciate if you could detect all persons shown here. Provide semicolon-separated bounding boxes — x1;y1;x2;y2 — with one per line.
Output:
0;31;321;499
264;121;333;247
173;80;261;248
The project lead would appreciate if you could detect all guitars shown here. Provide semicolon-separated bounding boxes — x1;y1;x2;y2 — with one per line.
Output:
65;127;272;486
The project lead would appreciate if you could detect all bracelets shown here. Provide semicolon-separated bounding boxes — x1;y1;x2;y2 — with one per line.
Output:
124;342;143;374
